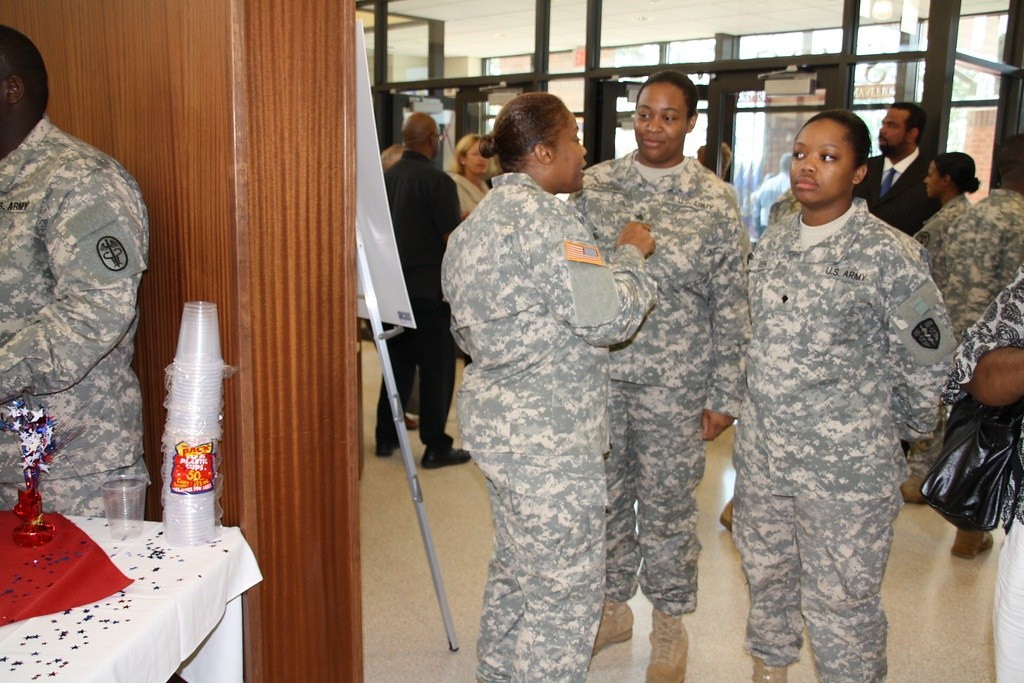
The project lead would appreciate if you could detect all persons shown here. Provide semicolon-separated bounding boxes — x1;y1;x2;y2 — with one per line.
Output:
381;143;418;432
0;24;151;518
447;134;491;223
854;102;944;236
899;134;1024;560
732;111;958;683
375;112;471;467
442;70;751;683
899;151;980;288
953;260;1024;683
752;152;791;247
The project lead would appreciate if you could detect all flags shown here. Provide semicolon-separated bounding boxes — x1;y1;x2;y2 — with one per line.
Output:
753;157;761;189
733;160;744;207
746;160;753;191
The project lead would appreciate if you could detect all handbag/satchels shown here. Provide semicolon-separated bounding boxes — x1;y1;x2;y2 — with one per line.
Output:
921;395;1024;530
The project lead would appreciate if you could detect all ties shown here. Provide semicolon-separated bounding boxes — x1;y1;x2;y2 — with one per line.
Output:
880;166;895;197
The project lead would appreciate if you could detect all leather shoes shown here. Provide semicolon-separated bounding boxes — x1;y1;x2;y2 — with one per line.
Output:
376;441;401;457
420;446;473;469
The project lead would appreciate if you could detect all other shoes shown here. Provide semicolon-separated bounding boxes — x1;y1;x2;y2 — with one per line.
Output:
950;524;993;559
403;414;421;430
900;478;927;505
718;503;733;528
753;655;787;683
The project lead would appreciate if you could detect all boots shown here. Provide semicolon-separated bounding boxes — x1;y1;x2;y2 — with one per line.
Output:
591;598;635;656
646;609;687;683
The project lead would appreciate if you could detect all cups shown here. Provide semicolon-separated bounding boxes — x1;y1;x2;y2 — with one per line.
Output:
100;475;148;541
162;301;223;548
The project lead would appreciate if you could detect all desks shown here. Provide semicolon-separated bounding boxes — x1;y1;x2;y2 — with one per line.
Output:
0;514;264;683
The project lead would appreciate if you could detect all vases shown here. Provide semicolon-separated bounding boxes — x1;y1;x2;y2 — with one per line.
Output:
11;478;55;548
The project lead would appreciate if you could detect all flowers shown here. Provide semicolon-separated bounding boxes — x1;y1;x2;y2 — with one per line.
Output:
0;394;67;476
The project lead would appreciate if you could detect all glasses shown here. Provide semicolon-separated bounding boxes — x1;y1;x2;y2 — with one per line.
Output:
427;132;443;142
